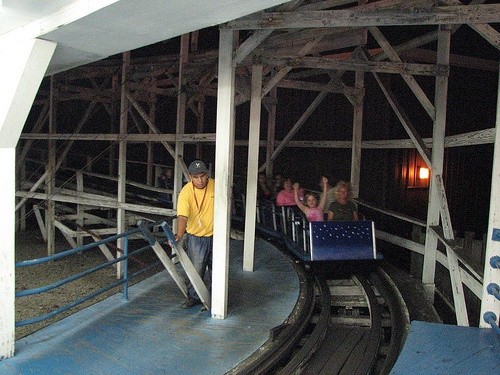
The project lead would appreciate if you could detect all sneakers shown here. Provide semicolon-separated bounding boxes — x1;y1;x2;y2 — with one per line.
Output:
182;298;202;308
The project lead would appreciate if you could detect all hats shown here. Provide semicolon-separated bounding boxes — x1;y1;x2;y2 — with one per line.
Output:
188;160;207;174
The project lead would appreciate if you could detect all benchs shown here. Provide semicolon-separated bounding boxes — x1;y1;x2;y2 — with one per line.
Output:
231;193;310;252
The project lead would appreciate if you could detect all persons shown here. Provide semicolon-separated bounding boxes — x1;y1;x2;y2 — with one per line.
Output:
159;170;357;222
168;160;214;311
